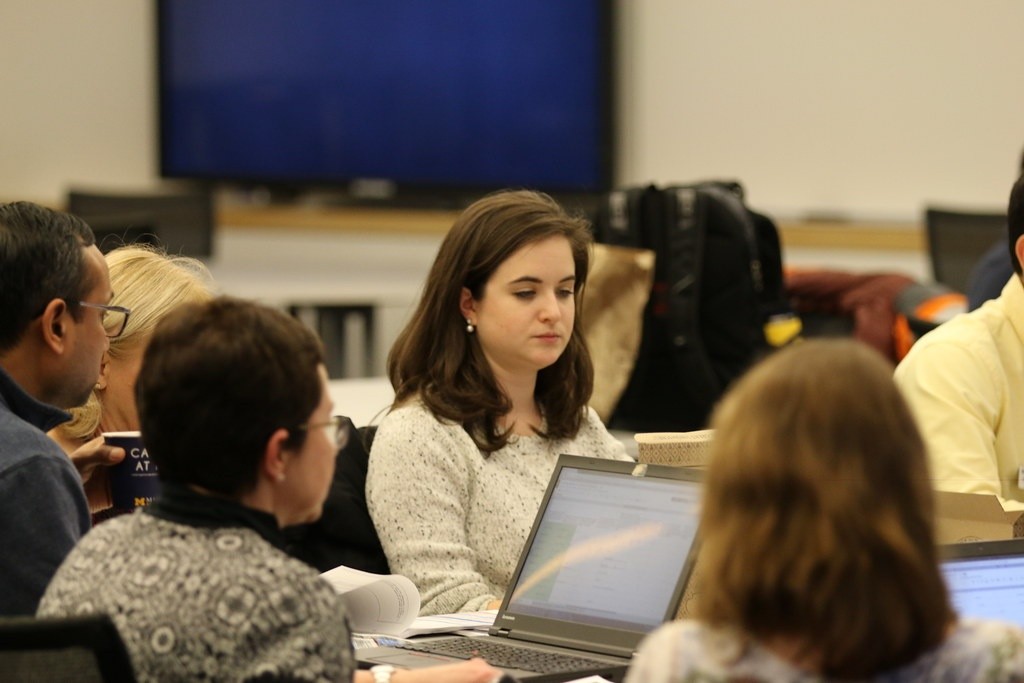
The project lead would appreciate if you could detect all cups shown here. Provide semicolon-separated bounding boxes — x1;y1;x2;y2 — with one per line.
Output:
101;431;158;516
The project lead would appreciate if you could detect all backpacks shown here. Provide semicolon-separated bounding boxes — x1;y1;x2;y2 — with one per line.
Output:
602;181;783;432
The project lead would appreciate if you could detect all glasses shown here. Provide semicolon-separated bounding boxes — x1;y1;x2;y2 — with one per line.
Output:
79;300;130;339
286;415;353;450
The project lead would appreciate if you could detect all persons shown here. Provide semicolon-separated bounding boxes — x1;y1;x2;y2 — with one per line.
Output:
0;197;131;617
32;293;502;683
47;244;220;527
891;173;1024;496
364;187;636;616
619;336;1024;683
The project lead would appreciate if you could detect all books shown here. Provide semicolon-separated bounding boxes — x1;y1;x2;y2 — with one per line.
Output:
311;563;499;640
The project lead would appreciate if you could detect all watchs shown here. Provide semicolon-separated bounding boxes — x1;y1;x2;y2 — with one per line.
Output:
369;663;395;683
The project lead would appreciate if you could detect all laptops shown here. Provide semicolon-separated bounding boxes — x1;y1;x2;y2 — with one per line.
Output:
349;455;716;683
926;535;1024;640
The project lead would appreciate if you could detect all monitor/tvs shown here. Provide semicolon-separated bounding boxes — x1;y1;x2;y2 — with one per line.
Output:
148;0;617;232
